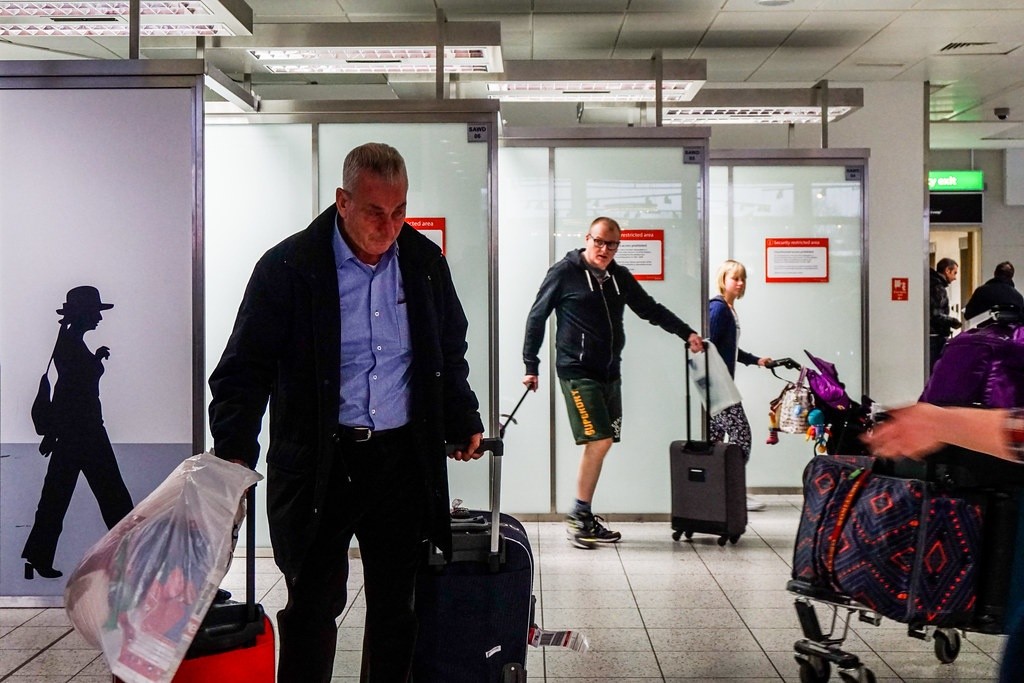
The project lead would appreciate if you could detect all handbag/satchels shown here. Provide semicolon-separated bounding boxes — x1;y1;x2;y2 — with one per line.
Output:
791;450;994;627
684;339;743;416
779;367;812;435
67;449;261;683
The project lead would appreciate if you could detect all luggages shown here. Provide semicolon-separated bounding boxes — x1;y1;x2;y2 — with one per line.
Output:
113;459;276;683
669;340;749;546
409;436;532;682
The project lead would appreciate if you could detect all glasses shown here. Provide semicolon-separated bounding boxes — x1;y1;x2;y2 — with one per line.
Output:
589;232;621;251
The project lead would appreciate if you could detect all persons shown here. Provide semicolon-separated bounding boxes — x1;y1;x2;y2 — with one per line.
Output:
709;260;765;511
964;261;1024;333
861;402;1024;464
522;217;705;549
930;258;962;371
209;143;485;683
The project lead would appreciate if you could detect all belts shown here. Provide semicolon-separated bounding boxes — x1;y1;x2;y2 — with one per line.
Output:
336;421;414;445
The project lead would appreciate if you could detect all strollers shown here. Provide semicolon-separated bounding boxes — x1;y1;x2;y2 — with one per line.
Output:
766;357;877;455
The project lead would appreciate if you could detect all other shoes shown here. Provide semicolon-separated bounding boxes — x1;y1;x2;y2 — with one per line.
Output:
746;497;766;510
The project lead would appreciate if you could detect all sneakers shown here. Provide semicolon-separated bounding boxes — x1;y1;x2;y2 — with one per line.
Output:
580;512;621;542
562;510;597;549
